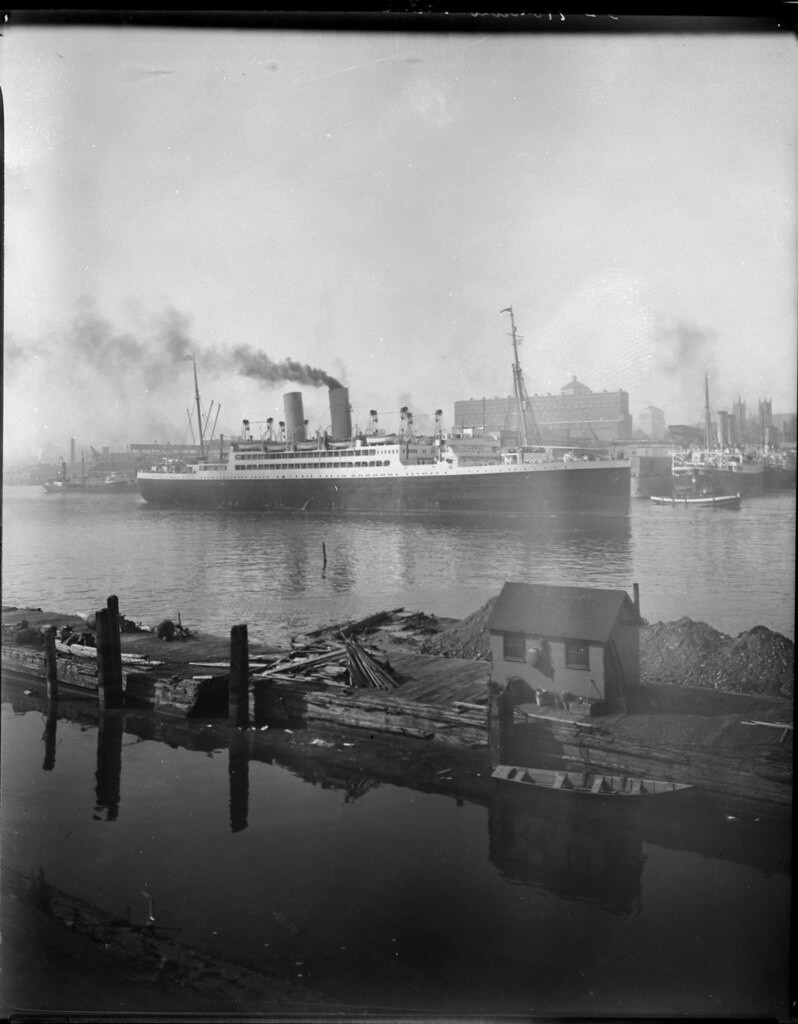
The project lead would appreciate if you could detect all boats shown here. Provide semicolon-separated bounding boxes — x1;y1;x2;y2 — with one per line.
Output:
134;307;632;515
649;493;742;506
39;473;134;493
491;762;695;812
671;448;796;485
